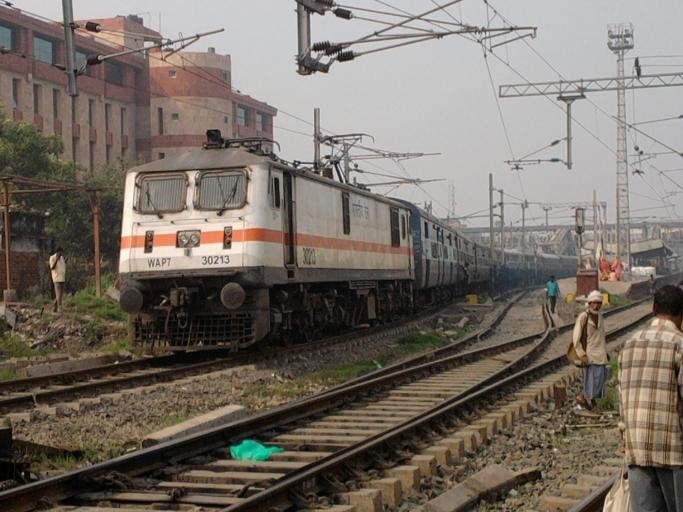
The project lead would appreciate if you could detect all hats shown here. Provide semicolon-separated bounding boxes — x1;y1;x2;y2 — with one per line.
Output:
586;289;604;303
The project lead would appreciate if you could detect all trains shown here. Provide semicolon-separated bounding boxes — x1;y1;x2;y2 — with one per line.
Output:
118;129;578;353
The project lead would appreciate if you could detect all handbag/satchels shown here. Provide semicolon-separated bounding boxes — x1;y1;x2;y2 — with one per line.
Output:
567;341;586;368
601;476;632;512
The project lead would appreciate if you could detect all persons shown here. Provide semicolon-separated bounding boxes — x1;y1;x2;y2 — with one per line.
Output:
572;289;610;409
546;275;560;313
49;246;66;315
618;284;683;512
599;255;623;282
